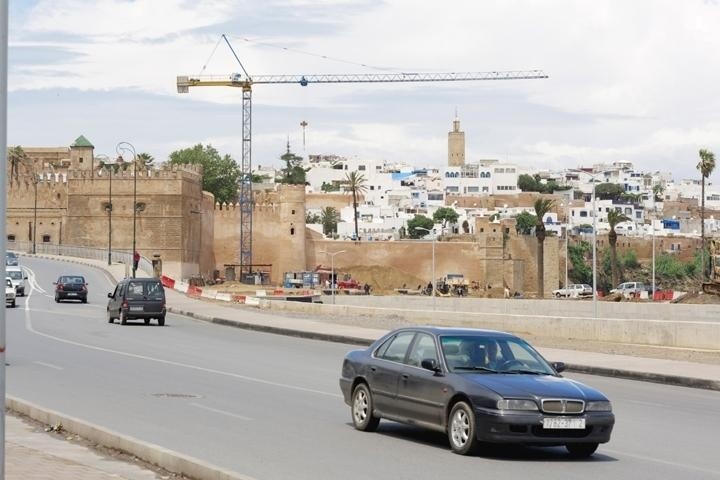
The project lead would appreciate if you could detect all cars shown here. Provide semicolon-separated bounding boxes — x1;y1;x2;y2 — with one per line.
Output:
5;251;28;308
552;283;593;298
644;285;661;298
52;275;88;303
339;326;615;457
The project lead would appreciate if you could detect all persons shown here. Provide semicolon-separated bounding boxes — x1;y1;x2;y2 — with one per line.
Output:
364;283;370;295
504;286;524;299
134;251;140;270
443;277;449;293
458;287;464;298
484;341;505;370
427;280;433;296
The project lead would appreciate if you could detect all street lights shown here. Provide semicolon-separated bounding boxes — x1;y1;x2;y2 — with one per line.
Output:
116;141;136;279
318;250;346;304
641;193;671;301
414;226;453;298
96;153;112;265
568;168;619;301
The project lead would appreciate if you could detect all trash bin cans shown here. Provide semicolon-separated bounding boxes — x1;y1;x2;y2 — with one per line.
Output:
214;270;219;280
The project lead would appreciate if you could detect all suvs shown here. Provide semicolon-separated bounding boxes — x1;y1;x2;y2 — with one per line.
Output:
106;278;166;326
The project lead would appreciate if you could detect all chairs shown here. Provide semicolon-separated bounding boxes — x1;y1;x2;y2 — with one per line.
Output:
441;344;477;371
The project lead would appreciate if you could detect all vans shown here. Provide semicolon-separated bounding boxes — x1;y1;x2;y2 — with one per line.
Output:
609;281;644;301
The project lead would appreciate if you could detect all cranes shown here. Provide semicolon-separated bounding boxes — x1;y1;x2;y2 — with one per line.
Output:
176;34;549;285
